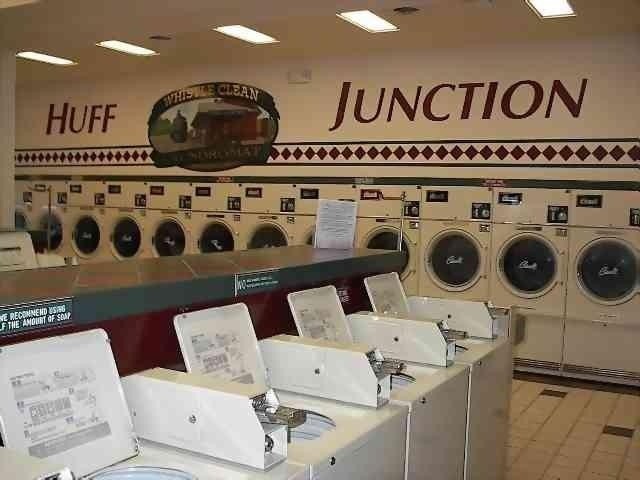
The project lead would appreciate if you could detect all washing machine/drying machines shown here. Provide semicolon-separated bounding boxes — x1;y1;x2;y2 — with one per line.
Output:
0;174;640;480
